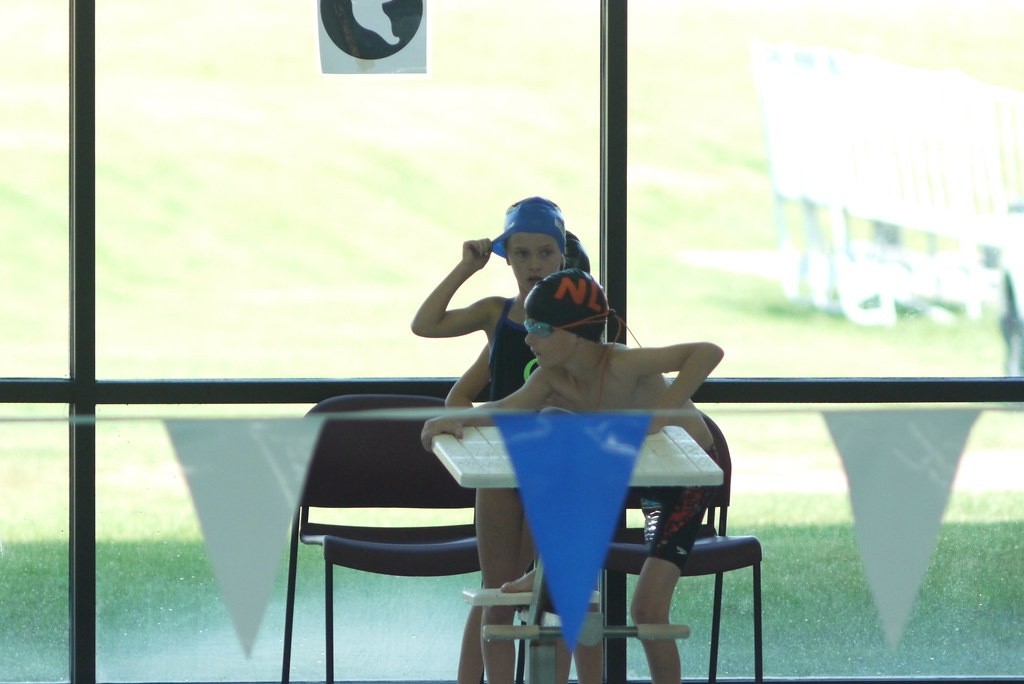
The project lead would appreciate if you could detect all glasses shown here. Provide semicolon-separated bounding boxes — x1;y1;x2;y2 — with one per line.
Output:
523;320;555;337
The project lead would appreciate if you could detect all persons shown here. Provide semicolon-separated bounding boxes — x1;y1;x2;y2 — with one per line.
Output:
421;270;733;684
411;197;604;684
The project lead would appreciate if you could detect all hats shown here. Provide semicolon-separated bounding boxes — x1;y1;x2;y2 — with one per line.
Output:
489;196;567;258
526;268;610;341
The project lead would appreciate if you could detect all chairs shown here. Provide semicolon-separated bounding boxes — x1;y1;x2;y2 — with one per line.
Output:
281;392;525;684
600;409;761;682
